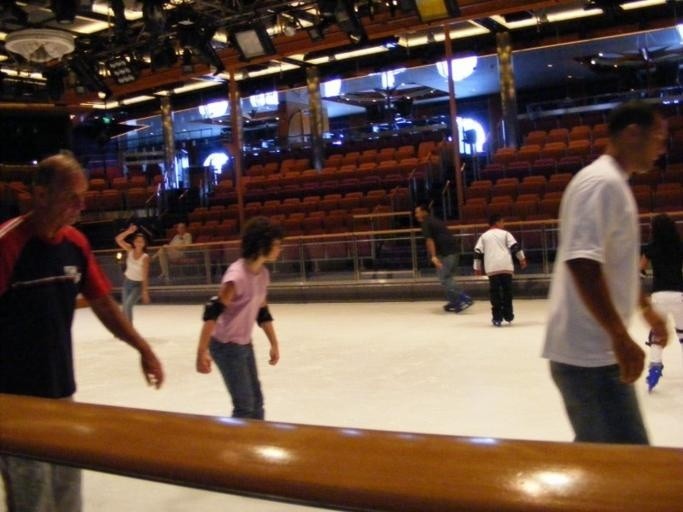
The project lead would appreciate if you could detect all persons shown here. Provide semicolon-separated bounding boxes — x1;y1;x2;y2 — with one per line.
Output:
113;223;152;330
196;217;285;421
413;205;473;313
639;212;682;389
0;151;164;511
150;220;193;282
472;213;529;325
540;99;670;445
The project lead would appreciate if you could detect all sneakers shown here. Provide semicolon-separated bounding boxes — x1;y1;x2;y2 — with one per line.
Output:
443;300;457;312
646;360;665;392
454;292;475;313
492;313;515;326
